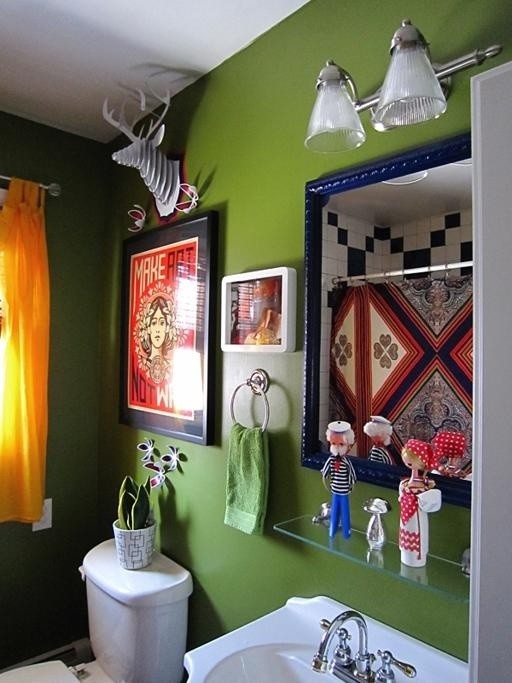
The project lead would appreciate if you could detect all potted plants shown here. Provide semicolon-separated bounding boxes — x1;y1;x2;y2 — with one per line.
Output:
111;475;158;571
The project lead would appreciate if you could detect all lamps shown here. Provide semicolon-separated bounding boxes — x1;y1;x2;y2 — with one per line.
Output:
304;17;449;156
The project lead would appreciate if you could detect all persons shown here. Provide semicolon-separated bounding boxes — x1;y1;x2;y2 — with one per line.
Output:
140;298;172;363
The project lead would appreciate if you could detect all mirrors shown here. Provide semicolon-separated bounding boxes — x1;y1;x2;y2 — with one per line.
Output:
300;131;472;510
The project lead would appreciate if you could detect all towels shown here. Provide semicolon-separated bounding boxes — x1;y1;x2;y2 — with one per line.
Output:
224;423;270;538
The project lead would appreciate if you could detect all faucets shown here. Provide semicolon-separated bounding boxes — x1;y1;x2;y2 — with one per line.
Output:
311;609;375;680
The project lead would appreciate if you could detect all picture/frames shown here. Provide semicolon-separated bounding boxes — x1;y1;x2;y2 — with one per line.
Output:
117;211;219;445
220;267;297;355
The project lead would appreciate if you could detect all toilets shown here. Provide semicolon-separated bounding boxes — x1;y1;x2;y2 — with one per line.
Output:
0;538;193;683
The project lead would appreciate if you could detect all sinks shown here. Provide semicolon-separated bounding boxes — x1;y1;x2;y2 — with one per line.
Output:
183;593;469;683
